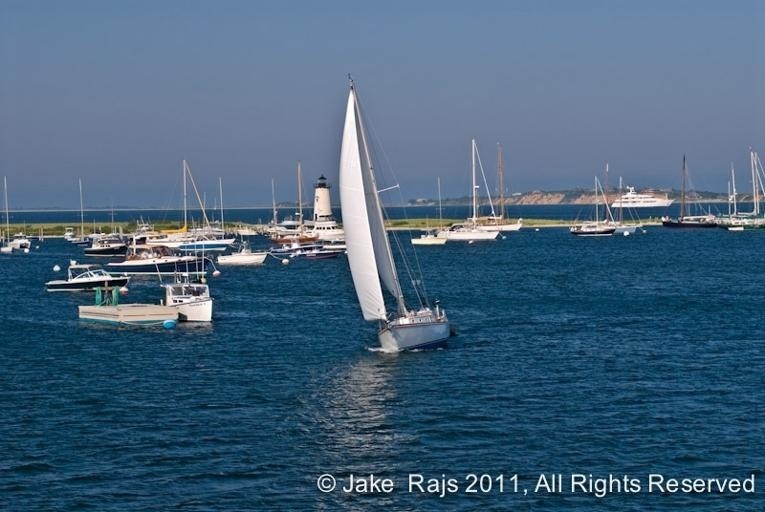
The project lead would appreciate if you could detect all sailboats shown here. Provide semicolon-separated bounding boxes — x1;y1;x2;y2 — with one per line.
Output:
661;148;765;232
570;163;651;237
63;160;345;258
410;139;523;245
338;73;451;353
1;176;15;255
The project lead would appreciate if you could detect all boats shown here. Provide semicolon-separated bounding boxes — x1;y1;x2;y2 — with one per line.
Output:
611;186;675;207
12;233;31;250
44;245;268;323
